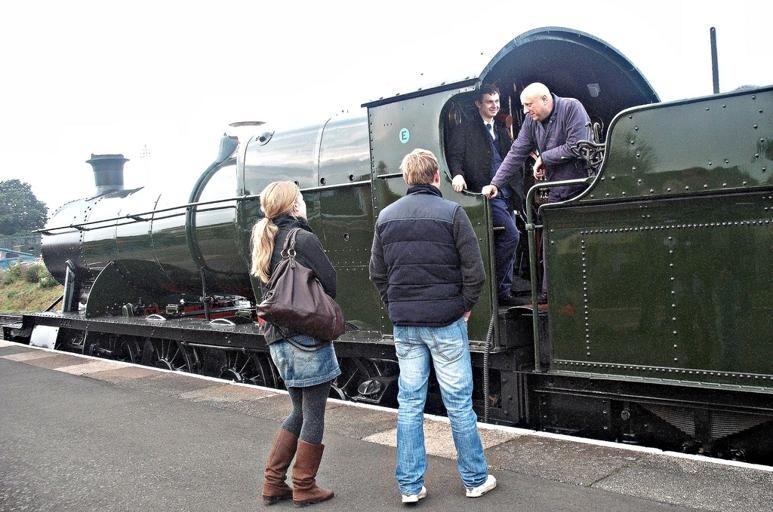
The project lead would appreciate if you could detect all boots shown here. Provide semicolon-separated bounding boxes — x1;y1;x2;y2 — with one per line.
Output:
291;440;334;506
262;428;299;506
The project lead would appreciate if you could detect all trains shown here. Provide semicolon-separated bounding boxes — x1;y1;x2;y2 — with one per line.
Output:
2;26;771;462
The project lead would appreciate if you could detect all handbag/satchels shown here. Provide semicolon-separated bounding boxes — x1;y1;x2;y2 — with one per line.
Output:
255;228;346;339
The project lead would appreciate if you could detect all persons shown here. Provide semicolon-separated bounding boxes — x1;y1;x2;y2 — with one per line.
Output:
248;179;334;506
368;147;497;503
447;84;524;303
482;82;597;305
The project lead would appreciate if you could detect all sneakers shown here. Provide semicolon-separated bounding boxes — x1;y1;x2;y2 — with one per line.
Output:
401;486;428;503
465;474;497;498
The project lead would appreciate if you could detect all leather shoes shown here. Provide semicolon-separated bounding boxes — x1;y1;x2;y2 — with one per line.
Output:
498;295;527;306
531;292;547;303
